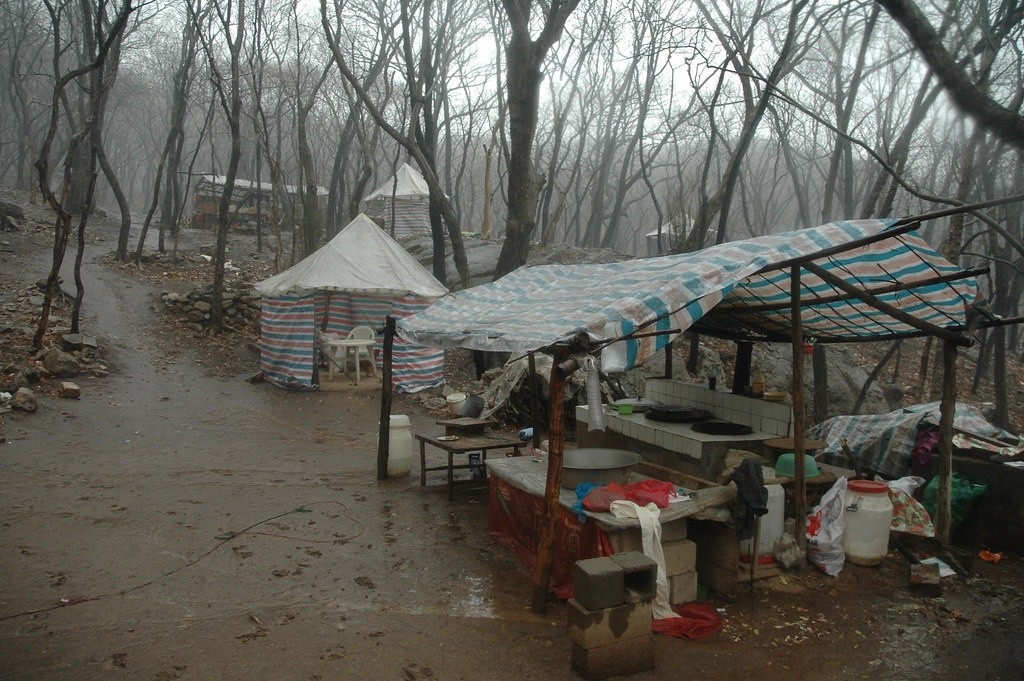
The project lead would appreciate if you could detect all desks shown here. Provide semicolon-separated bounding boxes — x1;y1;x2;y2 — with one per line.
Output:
329;339;377;384
415;433;527;502
776;462;868;520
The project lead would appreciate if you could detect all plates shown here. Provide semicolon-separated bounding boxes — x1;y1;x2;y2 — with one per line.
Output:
437;435;459;441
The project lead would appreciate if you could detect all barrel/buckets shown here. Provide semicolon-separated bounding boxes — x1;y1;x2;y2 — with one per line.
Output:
842;478;893;568
376;415;413;478
446;393;466;414
735;467;784;563
461;394;485;418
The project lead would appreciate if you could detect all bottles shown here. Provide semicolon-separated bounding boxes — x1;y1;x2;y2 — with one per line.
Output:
752;370;765;397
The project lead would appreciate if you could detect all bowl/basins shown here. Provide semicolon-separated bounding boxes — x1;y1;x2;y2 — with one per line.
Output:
774;453;820;478
618;404;633;414
763;392;789;401
542;448;641;490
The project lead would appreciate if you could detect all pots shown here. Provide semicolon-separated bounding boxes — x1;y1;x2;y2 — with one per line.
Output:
609;393;664;412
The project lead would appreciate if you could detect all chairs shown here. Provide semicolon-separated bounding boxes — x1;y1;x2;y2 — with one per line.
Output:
344;326;375;372
